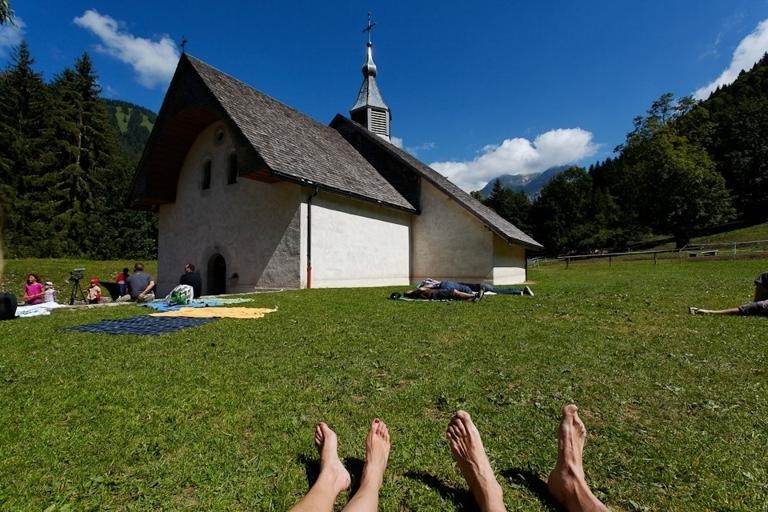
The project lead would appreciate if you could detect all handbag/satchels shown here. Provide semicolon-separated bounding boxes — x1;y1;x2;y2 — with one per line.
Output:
165;284;195;306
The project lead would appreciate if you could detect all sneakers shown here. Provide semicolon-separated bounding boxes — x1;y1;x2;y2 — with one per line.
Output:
687;305;698;316
512;285;535;297
473;289;484;304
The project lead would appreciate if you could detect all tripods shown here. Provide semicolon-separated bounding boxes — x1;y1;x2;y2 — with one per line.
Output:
68;281;88;305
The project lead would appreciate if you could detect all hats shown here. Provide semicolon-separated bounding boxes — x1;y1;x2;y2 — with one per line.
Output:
89;278;99;285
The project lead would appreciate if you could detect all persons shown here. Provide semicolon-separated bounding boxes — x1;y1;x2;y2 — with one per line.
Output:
285;417;392;512
23;273;46;306
403;278;534;303
116;267;130;297
445;404;610;512
125;262;155;302
688;272;768;317
179;263;202;298
86;278;101;304
43;281;57;303
